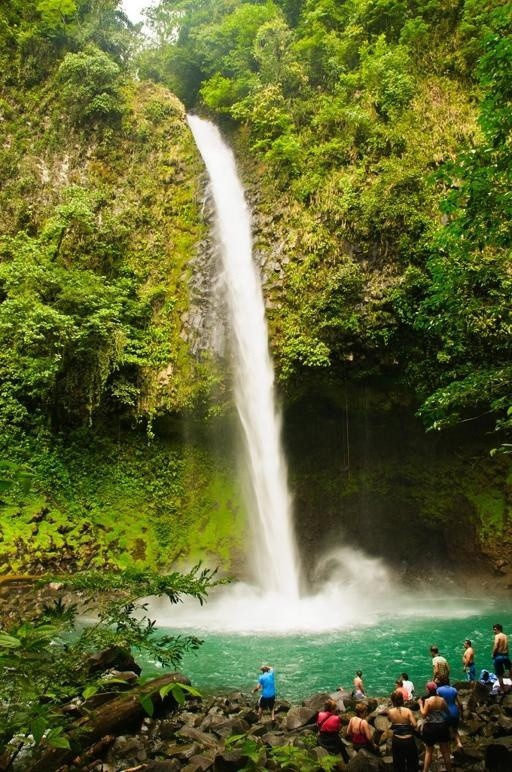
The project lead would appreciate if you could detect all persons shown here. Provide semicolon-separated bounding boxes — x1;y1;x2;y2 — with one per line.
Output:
252;666;276;724
318;623;512;772
